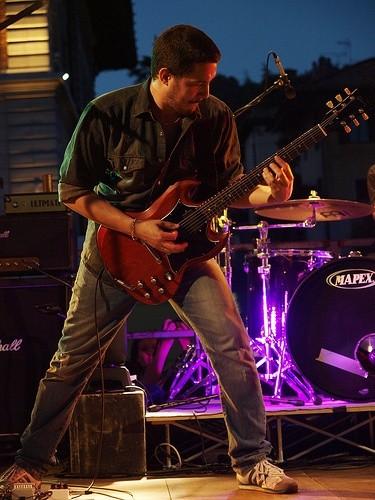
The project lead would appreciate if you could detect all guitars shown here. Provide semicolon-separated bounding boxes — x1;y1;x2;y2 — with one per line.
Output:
95;85;369;306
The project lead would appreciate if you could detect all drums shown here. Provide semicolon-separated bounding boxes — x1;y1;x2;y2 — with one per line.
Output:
284;253;375;405
240;248;332;340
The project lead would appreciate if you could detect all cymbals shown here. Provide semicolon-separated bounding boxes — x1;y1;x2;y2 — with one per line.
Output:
254;196;372;222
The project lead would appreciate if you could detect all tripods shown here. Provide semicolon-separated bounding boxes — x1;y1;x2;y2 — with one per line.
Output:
231;205;323;405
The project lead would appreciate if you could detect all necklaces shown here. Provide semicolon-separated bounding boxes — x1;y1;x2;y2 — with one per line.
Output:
161;117;180;125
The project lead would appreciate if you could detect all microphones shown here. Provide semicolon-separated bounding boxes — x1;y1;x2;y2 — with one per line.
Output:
272;52;296;99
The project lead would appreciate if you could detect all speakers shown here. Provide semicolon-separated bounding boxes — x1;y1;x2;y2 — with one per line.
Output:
0;275;75;444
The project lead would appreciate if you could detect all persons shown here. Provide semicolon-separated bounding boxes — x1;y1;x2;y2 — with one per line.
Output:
0;24;299;500
134;318;197;406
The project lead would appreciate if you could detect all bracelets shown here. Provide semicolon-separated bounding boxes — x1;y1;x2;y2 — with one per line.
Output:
131;218;138;242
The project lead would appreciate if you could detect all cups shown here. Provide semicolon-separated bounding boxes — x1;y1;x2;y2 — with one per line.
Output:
42;174;53;192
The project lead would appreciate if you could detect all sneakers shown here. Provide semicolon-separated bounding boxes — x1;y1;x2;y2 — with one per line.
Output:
236;458;298;493
0;464;41;500
